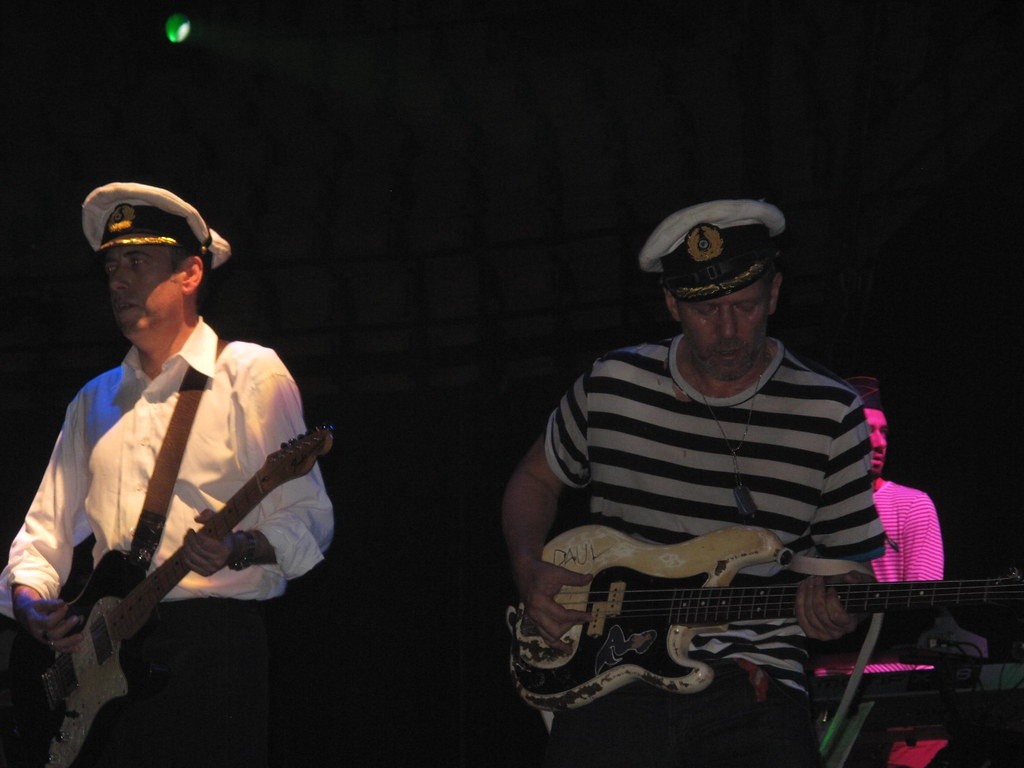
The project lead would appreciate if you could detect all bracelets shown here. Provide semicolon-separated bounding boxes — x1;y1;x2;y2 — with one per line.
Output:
229;531;255;570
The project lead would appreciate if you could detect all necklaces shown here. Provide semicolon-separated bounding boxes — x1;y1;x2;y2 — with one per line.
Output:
688;358;763;516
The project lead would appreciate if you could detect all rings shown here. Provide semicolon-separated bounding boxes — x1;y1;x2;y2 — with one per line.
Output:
49;640;54;647
43;632;49;640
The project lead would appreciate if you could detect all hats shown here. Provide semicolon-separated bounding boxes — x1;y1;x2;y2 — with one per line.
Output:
847;377;884;411
82;181;231;268
639;199;786;303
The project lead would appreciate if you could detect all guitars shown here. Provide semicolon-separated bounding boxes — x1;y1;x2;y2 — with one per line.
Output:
505;523;1024;713
6;420;335;768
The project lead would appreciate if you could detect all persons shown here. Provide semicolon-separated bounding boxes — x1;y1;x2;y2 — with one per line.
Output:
501;200;886;768
813;377;944;768
0;183;335;768
